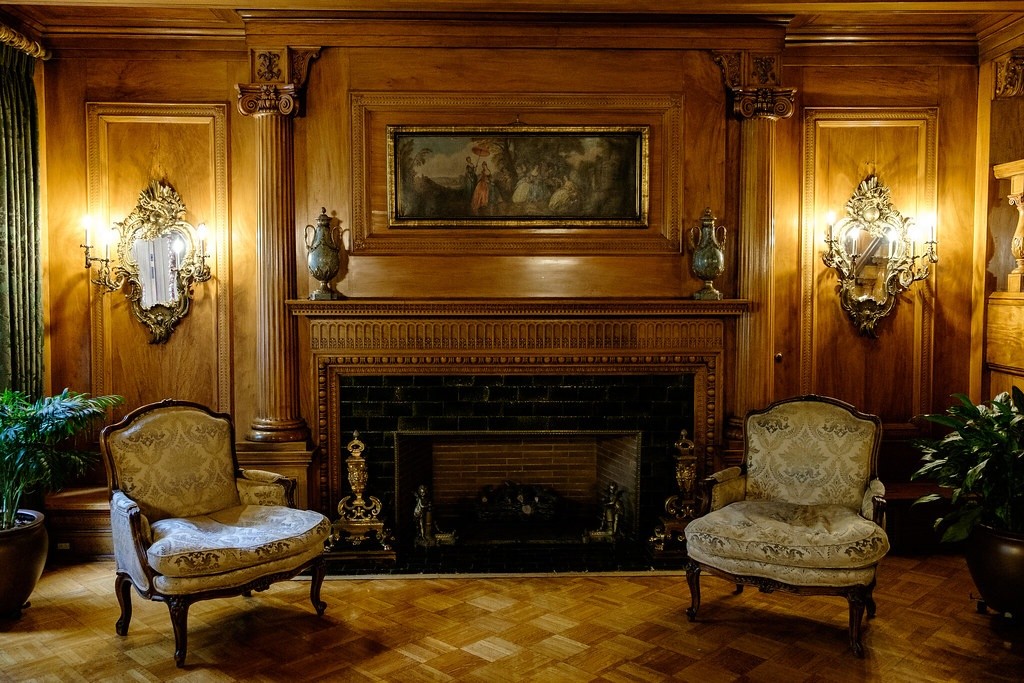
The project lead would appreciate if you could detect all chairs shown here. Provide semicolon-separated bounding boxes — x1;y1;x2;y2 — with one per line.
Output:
662;393;889;653
100;399;336;669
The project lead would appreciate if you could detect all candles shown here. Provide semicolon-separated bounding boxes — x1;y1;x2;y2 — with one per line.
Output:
824;210;835;239
81;214;91;246
909;227;920;256
103;232;115;259
849;227;859;255
887;231;895;258
197;224;208;255
173;238;183;268
926;213;935;241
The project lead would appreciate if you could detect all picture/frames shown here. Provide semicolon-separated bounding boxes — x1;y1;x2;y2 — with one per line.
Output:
387;124;649;228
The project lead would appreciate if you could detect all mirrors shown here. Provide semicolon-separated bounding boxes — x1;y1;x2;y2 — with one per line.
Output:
90;178;212;345
823;179;930;341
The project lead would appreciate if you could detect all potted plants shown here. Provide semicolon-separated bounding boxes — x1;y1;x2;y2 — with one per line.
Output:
908;385;1024;620
0;389;125;632
